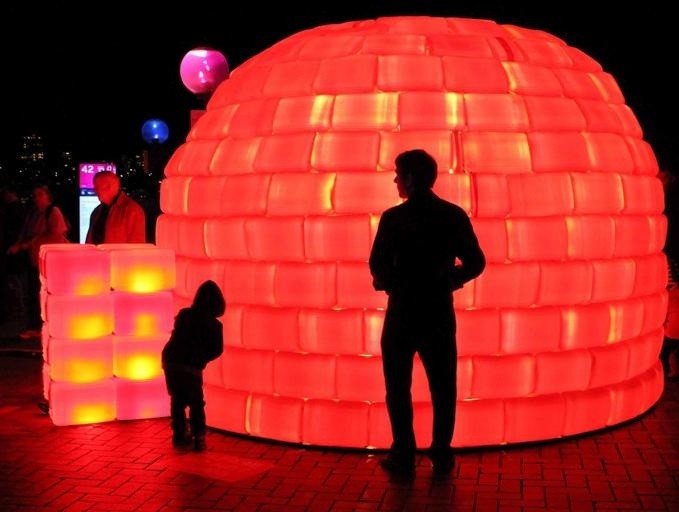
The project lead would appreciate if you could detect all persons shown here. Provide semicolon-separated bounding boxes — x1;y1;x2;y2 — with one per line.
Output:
2;185;71;341
82;171;147;245
0;189;29;247
160;278;225;452
658;275;679;383
367;147;489;478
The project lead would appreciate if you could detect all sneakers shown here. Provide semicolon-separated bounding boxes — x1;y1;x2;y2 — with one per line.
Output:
173;431;205;449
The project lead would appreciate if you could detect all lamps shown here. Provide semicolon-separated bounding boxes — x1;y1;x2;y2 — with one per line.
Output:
141;119;169;177
179;47;230;130
